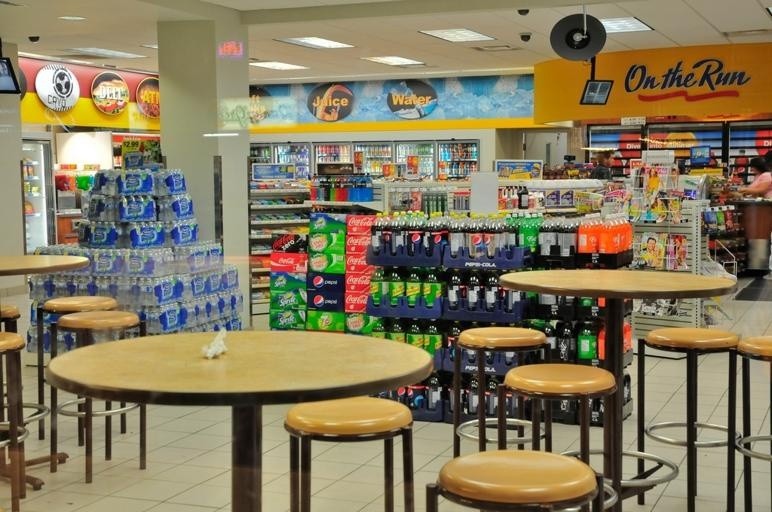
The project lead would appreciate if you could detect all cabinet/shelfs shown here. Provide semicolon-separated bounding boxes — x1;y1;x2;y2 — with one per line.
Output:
20;116;772;340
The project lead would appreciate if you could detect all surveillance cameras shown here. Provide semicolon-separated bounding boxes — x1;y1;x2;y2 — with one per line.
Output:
517;9;529;16
29;36;39;42
519;32;532;42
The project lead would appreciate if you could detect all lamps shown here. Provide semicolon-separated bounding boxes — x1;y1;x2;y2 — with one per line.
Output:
550;0;614;106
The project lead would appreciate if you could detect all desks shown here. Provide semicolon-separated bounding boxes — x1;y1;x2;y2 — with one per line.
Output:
0;254;90;490
500;264;736;511
45;330;433;512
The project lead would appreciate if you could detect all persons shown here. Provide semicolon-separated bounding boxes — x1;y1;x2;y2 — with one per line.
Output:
588;150;614;181
765;150;772;172
738;156;772;200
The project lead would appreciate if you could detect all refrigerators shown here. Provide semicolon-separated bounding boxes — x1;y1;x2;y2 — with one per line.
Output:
20;131;58;255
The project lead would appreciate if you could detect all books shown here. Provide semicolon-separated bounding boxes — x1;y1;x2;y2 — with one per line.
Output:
630;232;688;270
659;193;660;194
631;164;684;222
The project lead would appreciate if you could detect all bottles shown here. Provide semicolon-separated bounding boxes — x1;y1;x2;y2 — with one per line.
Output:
367;209;634;422
734;150;750;184
279;144;478;201
498;186;529;209
26;168;244;354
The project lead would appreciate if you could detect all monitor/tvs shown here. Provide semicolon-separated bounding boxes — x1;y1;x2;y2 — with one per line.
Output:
690;145;711;166
580;80;614;105
0;57;21;94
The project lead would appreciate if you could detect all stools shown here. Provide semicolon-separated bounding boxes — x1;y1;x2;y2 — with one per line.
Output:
499;363;619;510
453;325;551;460
0;296;160;512
726;337;772;511
284;398;414;512
637;327;752;510
426;449;605;510
